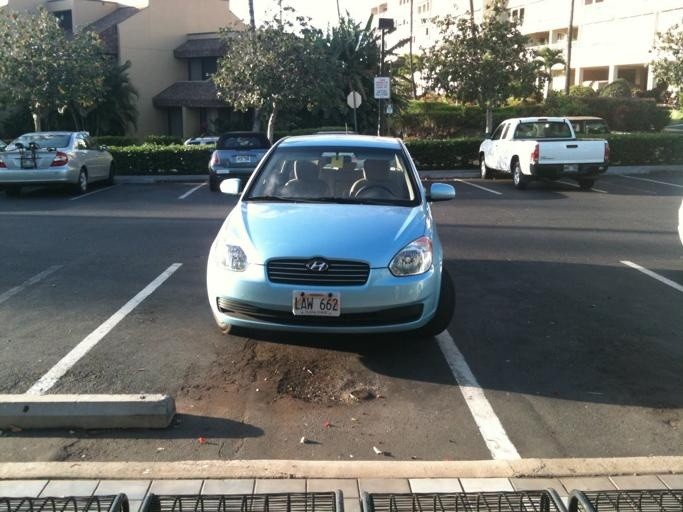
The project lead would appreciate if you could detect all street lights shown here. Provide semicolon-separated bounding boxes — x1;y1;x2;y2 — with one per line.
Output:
378;18;394;133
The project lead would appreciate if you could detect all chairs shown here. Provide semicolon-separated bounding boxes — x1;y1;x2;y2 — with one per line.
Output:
286;160;329;198
350;159;401;199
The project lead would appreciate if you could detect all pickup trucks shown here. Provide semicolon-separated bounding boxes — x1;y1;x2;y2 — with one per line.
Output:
478;115;613;190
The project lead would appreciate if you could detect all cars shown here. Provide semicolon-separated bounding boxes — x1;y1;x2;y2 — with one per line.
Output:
206;134;457;339
0;130;114;194
183;133;218;145
207;131;272;192
